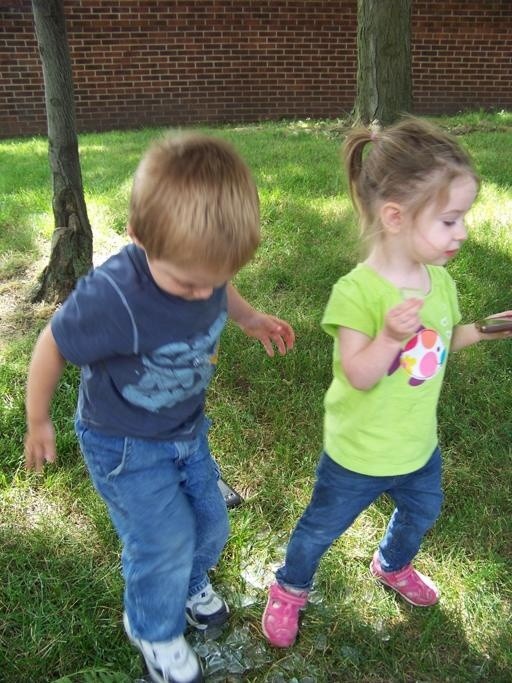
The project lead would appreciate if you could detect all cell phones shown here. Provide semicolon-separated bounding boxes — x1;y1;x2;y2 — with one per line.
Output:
478;315;512;332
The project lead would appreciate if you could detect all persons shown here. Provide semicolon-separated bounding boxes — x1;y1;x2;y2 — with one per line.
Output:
21;128;298;680
253;109;511;647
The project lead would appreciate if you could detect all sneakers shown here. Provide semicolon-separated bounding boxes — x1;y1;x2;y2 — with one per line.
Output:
185;583;230;630
369;550;440;607
261;582;308;648
122;600;205;683
